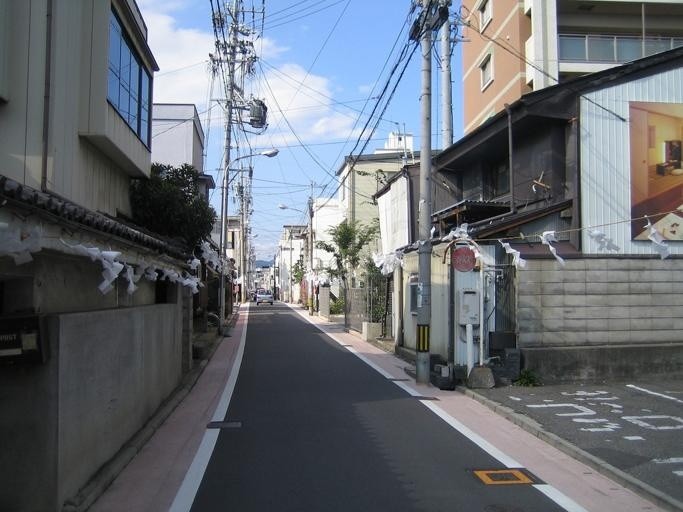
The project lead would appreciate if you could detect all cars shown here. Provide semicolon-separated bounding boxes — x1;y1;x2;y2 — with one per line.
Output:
254;287;274;307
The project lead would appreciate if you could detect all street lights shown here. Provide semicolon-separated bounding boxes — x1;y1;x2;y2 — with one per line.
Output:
241;232;258;303
219;147;280;328
278;203;313;316
